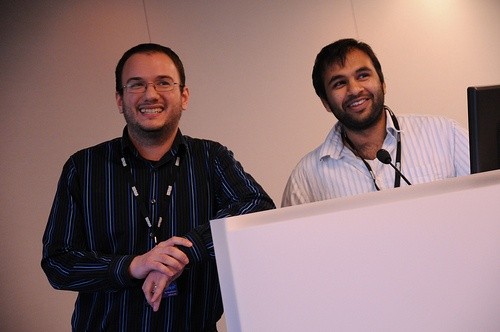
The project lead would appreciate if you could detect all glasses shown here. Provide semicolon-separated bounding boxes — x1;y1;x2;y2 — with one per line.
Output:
122;80;180;93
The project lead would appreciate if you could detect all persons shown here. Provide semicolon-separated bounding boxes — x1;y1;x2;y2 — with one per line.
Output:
41;43;278;332
279;39;470;207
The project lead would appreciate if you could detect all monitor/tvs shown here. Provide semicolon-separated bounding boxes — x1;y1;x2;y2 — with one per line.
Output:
467;84;500;175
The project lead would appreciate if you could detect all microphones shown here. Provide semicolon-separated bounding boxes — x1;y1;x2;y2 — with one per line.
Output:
376;149;411;186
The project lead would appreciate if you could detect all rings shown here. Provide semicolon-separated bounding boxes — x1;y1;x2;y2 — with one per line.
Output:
150;281;158;289
